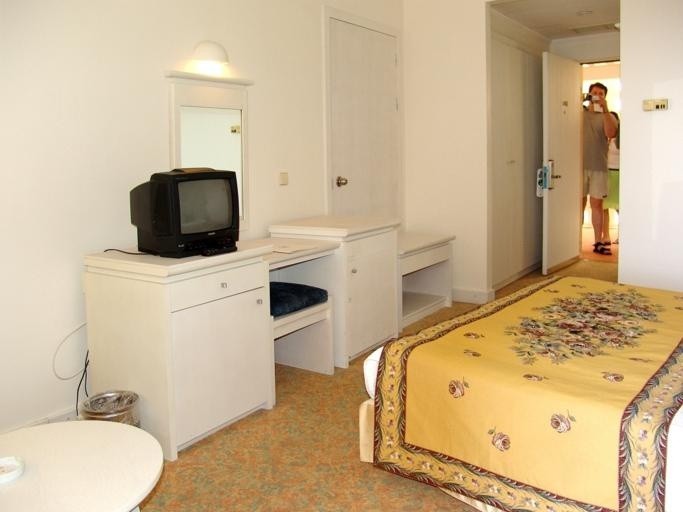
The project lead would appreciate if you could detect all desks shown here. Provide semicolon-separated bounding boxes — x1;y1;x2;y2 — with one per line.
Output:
0;420;164;512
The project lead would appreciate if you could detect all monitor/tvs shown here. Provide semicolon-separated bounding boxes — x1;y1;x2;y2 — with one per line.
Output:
129;168;239;258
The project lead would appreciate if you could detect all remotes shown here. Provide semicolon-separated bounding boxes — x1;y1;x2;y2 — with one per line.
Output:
200;244;237;256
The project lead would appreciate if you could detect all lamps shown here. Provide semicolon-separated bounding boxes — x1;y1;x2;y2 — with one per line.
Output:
191;38;228;64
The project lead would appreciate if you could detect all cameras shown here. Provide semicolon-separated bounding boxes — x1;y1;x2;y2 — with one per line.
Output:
582;93;593;108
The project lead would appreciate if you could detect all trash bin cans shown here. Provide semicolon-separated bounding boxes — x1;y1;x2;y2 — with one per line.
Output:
80;390;141;428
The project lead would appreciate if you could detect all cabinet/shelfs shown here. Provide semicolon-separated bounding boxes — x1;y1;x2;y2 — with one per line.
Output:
395;228;457;332
78;249;275;460
267;212;404;371
491;32;543;290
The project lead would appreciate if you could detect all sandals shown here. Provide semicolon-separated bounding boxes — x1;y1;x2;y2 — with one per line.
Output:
593;241;612;255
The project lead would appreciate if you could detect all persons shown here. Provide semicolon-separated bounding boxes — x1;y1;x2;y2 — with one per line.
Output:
603;112;619;244
582;83;616;253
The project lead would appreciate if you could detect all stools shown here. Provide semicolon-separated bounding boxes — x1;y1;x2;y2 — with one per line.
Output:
268;280;335;406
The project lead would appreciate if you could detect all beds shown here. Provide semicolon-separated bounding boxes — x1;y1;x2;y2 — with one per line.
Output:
359;269;683;512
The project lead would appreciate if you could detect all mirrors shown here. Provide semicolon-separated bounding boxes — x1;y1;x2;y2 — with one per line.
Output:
166;69;256;226
